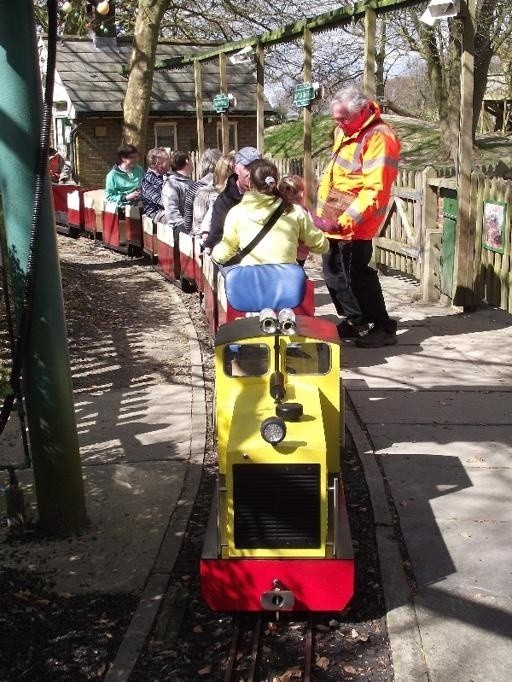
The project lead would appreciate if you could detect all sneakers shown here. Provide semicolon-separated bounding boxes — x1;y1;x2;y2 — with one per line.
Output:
356;326;397;347
336;318;368;338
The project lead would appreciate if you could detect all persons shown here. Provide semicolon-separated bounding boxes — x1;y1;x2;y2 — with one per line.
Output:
182;148;224;234
105;144;147;221
142;147;173;227
210;160;330;276
313;86;402;347
189;150;237;237
204;146;264;256
276;174;353;270
160;150;198;234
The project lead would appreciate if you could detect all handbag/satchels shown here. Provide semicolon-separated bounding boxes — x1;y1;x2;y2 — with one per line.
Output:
319;131;357;233
222;200;289;267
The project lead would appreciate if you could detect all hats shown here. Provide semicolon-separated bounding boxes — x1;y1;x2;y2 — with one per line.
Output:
234;147;262;165
